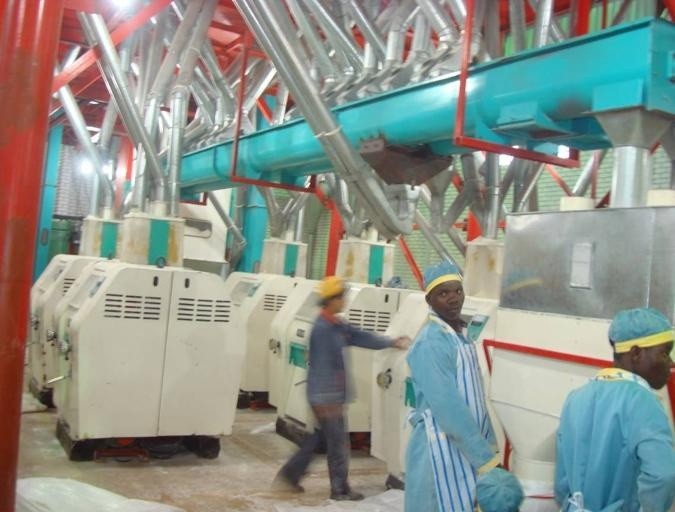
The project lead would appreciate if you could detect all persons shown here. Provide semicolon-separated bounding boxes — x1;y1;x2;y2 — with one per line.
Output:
553;308;675;512
405;261;500;512
271;275;413;501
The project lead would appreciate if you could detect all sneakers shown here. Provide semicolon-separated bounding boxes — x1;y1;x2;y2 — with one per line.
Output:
275;471;304;494
330;488;363;500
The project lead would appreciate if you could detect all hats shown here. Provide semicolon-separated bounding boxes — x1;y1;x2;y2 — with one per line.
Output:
606;308;673;353
421;263;460;295
317;278;344;298
